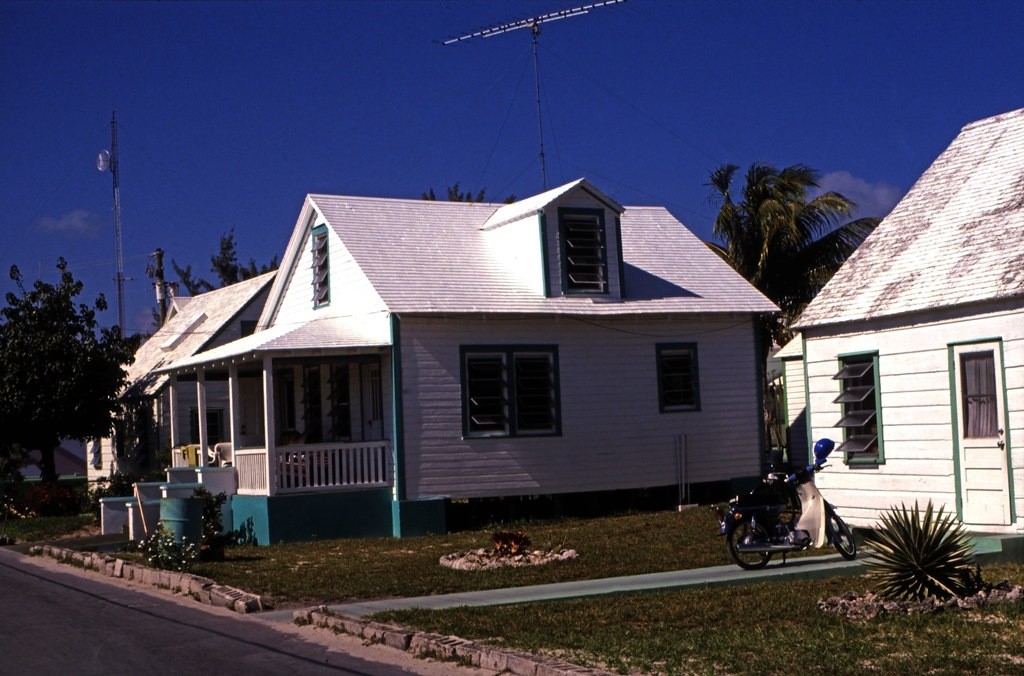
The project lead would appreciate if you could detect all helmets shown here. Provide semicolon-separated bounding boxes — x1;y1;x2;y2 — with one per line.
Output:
814;439;834;464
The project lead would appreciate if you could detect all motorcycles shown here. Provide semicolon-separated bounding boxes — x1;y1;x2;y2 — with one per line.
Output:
707;439;857;570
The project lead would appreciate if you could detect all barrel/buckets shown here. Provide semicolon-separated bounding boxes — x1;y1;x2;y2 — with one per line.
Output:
159;498;204;560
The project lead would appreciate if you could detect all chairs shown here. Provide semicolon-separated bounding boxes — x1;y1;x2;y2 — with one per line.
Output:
187;441;232;467
